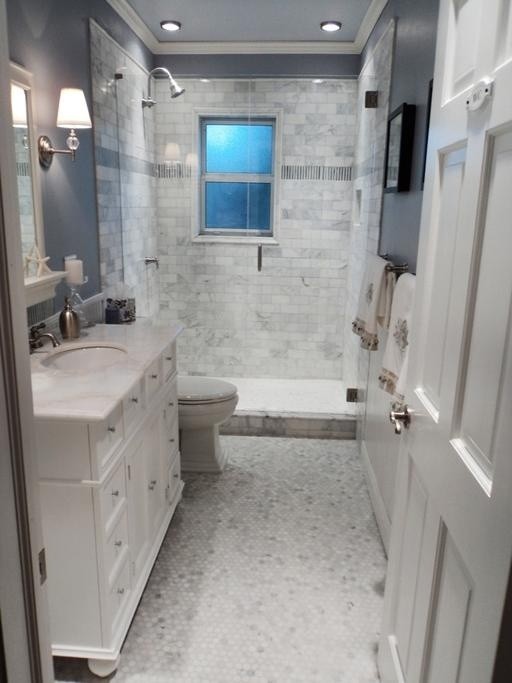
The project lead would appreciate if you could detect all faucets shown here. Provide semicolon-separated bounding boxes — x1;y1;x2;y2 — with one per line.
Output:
35;331;61;348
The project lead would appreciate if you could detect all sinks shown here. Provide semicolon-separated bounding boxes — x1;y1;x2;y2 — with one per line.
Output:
40;344;128;373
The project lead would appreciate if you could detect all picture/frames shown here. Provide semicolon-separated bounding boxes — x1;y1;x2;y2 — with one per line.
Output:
381;101;417;194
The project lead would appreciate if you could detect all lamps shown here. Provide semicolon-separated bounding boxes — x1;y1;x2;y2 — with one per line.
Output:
35;88;93;169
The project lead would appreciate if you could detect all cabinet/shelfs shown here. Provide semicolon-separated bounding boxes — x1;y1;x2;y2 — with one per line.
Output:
37;339;186;679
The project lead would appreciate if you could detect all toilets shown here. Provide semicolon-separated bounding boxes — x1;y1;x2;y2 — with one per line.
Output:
177;377;239;473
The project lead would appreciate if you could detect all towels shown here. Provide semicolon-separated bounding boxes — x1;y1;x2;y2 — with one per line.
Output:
350;249;417;406
